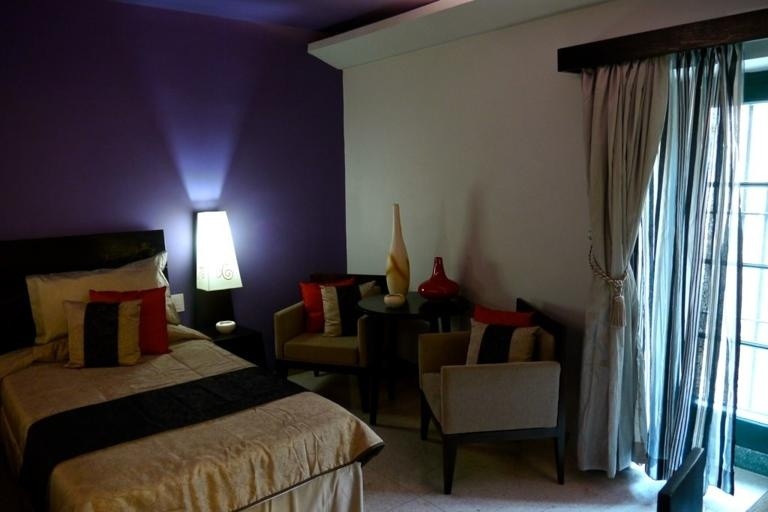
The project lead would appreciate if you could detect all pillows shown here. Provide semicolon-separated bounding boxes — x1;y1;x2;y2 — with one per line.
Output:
295;276;381;338
25;248;177;368
466;303;541;364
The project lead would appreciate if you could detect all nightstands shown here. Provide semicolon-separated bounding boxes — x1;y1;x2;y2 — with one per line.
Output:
196;324;256;365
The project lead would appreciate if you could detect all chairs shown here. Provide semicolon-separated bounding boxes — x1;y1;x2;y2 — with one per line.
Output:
272;272;389;411
416;297;569;496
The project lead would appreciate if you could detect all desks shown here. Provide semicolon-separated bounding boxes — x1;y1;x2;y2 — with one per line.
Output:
358;291;474;441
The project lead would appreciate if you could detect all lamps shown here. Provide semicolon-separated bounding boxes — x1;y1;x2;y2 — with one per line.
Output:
194;211;244;293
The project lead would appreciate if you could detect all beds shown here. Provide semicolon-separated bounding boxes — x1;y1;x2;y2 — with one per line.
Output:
2;230;367;512
